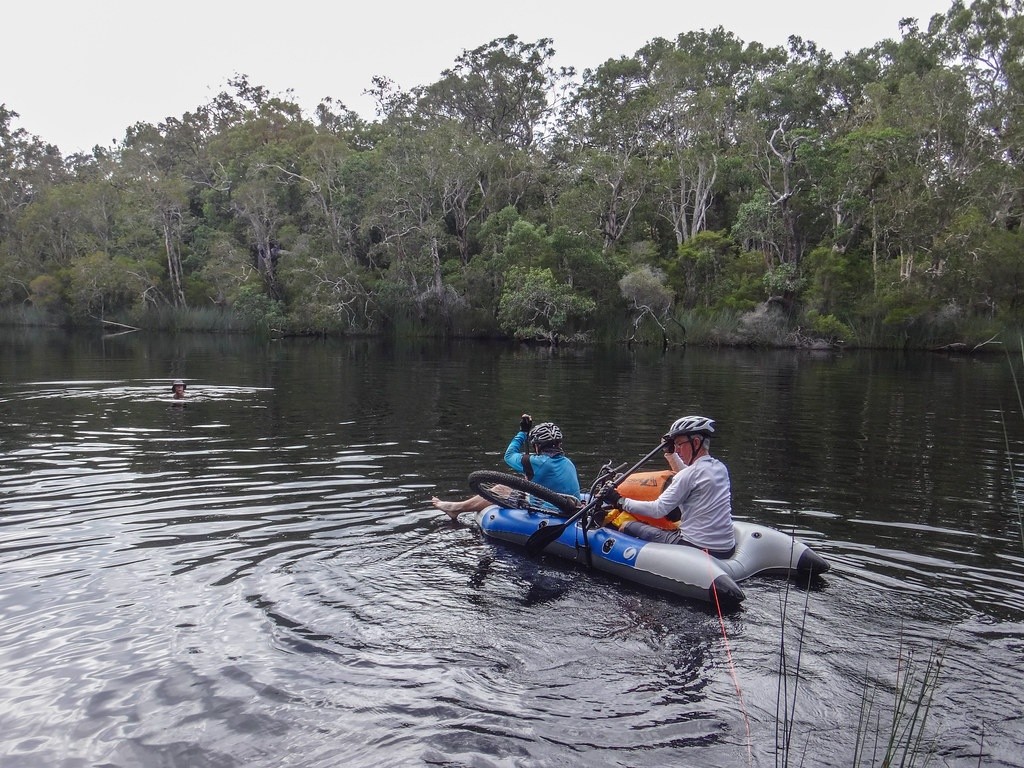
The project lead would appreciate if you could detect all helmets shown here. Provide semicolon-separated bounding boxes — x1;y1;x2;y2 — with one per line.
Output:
663;416;715;441
529;422;563;449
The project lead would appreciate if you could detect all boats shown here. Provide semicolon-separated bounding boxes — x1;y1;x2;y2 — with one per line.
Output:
475;492;831;605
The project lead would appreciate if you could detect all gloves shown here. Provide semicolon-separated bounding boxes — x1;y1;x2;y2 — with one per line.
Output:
520;414;532;434
594;484;623;510
661;438;675;454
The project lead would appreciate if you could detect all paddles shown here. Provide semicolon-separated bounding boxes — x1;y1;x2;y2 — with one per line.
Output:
521;443;668;560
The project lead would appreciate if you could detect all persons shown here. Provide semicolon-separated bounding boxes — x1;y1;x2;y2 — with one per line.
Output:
432;414;581;519
171;380;187;398
595;416;735;559
